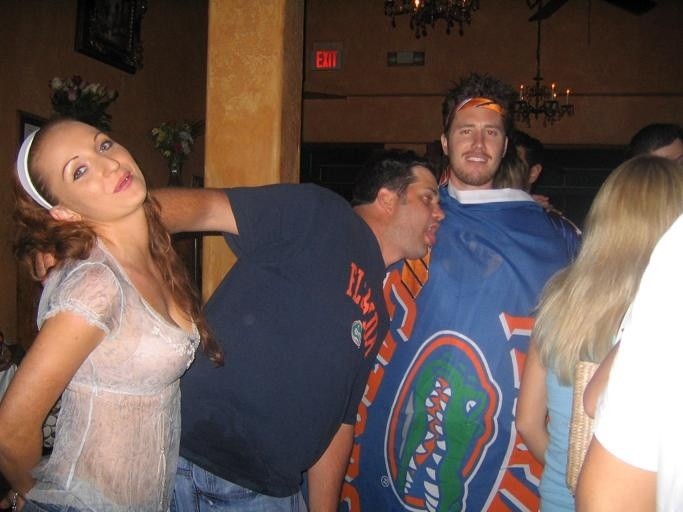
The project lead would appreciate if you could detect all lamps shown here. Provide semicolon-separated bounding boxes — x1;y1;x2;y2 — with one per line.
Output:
382;0;480;38
507;0;576;126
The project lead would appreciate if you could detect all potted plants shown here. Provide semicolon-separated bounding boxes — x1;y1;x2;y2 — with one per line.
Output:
150;116;210;171
49;78;116;129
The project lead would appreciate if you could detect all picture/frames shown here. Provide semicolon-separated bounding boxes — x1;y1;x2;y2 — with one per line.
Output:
14;108;47;147
74;0;149;75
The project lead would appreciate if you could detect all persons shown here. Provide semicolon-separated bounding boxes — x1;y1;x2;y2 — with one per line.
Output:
515;124;683;512
27;148;445;512
0;117;224;512
301;71;582;512
